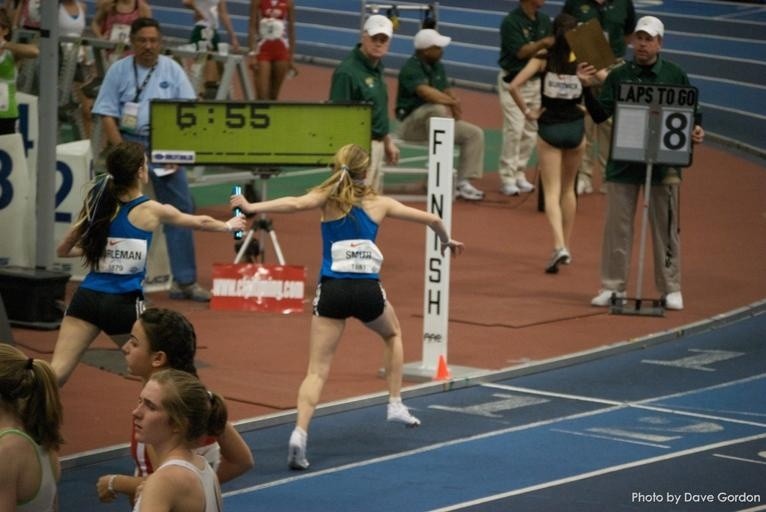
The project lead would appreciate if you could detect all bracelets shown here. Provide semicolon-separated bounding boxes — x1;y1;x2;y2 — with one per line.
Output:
108;475;119;499
442;238;450;246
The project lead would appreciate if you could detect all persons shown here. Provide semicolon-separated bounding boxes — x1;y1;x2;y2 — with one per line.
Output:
54;141;247;386
578;16;704;315
509;17;589;274
232;145;464;472
96;307;253;502
133;369;227;512
0;343;67;512
0;1;636;191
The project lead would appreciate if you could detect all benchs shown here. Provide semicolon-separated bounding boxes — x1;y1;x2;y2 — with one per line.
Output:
190;140;460;203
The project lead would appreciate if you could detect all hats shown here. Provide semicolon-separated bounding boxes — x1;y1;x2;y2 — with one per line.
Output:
362;13;394;39
414;28;450;51
637;14;664;40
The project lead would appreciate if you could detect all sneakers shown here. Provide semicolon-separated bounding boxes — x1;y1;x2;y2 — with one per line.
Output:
389;403;419;426
455;174;607;200
289;429;310;470
546;248;569;274
592;286;629;305
170;281;212;301
663;291;685;313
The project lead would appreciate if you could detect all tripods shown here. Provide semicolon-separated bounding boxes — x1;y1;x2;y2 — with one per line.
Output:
233;175;286;266
